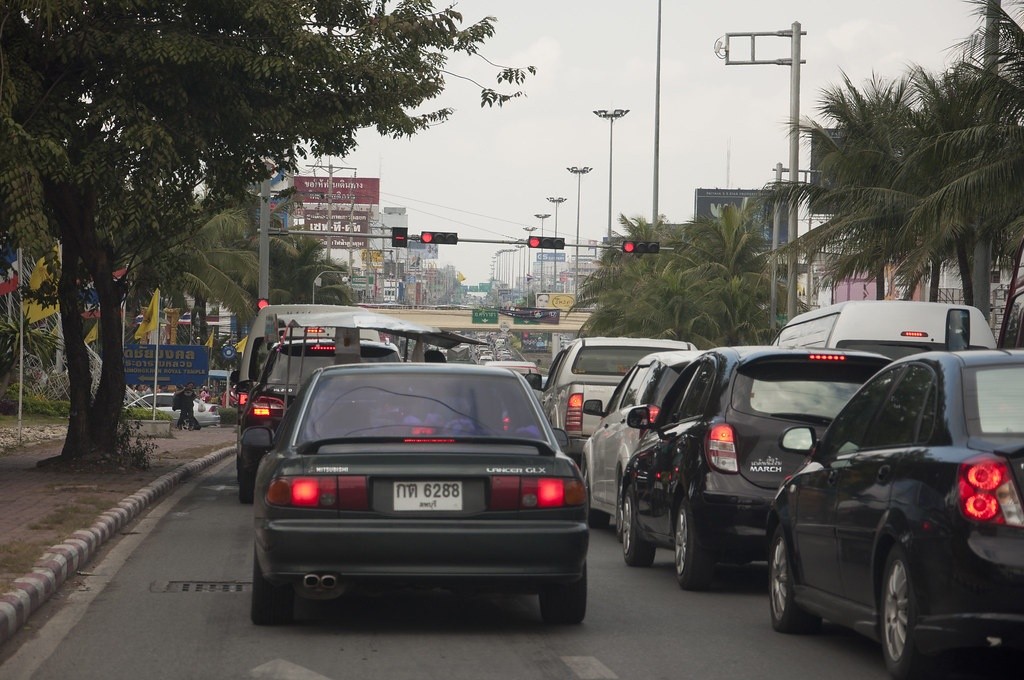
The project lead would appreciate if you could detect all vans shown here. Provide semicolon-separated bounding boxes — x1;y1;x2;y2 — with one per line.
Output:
484;360;539;397
230;303;397;424
757;301;999;428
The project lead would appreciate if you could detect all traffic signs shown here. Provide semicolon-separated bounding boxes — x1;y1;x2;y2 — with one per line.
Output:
472;307;499;324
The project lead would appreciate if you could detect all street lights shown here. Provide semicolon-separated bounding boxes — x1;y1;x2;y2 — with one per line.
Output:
523;226;538;309
514;244;525;298
534;213;551;294
592;109;631;245
546;197;567;293
312;270;351;305
510;248;518;300
567;166;593;302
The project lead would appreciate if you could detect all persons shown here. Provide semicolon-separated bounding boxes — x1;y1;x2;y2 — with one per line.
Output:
537;294;550;308
124;384;168;407
175;382;196;431
524;337;545;347
200;385;239;408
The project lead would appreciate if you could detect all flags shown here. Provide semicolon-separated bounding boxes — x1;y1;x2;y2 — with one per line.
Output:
135;314;143;325
134;288;159;340
176;313;191;325
233;337;247;353
23;244;60;324
84;323;97;344
81;269;127;318
0;240;18;295
205;333;213;347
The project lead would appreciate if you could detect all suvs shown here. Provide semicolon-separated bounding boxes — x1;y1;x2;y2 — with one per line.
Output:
525;336;699;467
236;337;411;505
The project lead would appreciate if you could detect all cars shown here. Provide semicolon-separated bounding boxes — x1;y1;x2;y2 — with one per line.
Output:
124;392;221;431
620;346;897;594
426;332;569;365
581;350;708;545
764;349;1024;680
240;361;590;627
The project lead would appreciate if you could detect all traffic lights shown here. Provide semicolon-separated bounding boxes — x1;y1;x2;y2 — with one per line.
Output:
420;231;457;244
391;227;408;247
257;297;271;312
622;241;659;253
529;236;565;249
